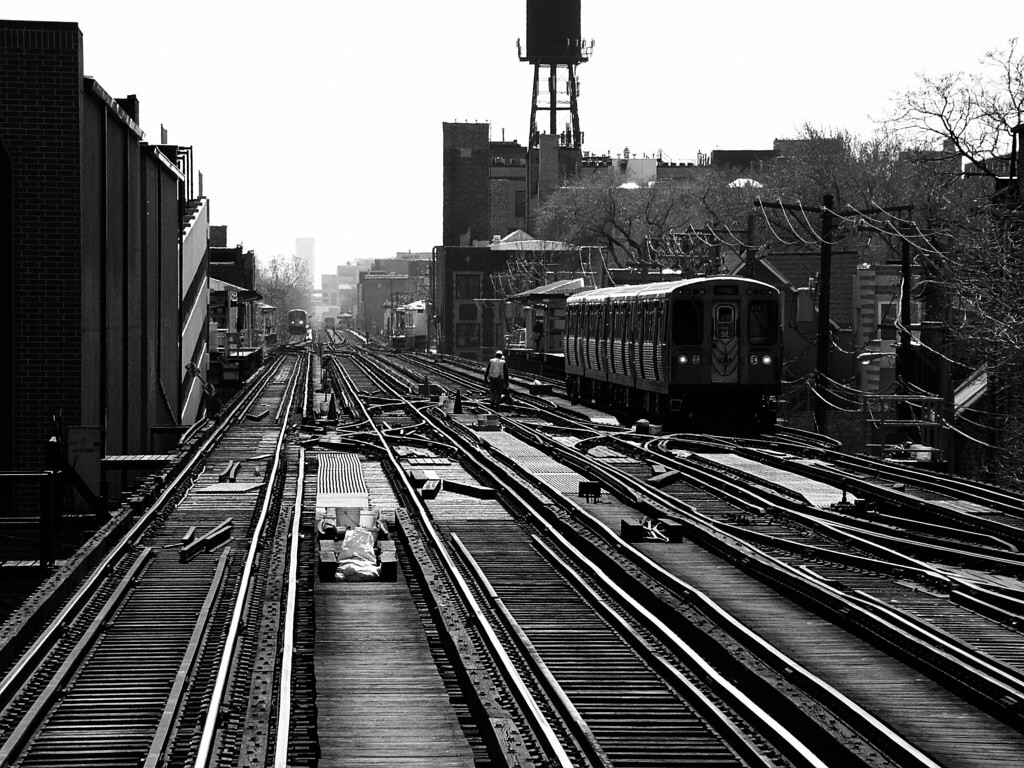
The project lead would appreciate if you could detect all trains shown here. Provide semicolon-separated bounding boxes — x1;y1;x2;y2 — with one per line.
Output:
562;274;786;437
287;308;310;336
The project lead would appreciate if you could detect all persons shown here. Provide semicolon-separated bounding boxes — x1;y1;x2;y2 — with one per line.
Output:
484;350;513;412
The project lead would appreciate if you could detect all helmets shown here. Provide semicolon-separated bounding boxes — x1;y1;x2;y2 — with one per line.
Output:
495;350;503;355
